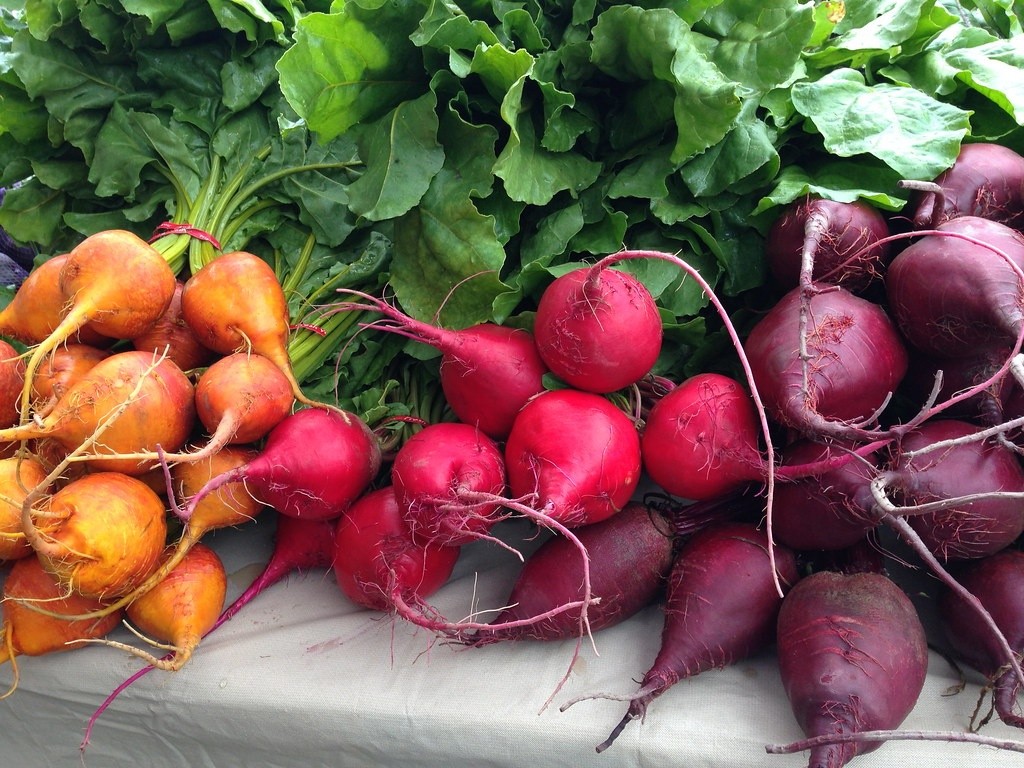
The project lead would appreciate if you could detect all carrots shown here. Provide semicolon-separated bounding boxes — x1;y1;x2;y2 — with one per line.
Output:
2;224;302;676
